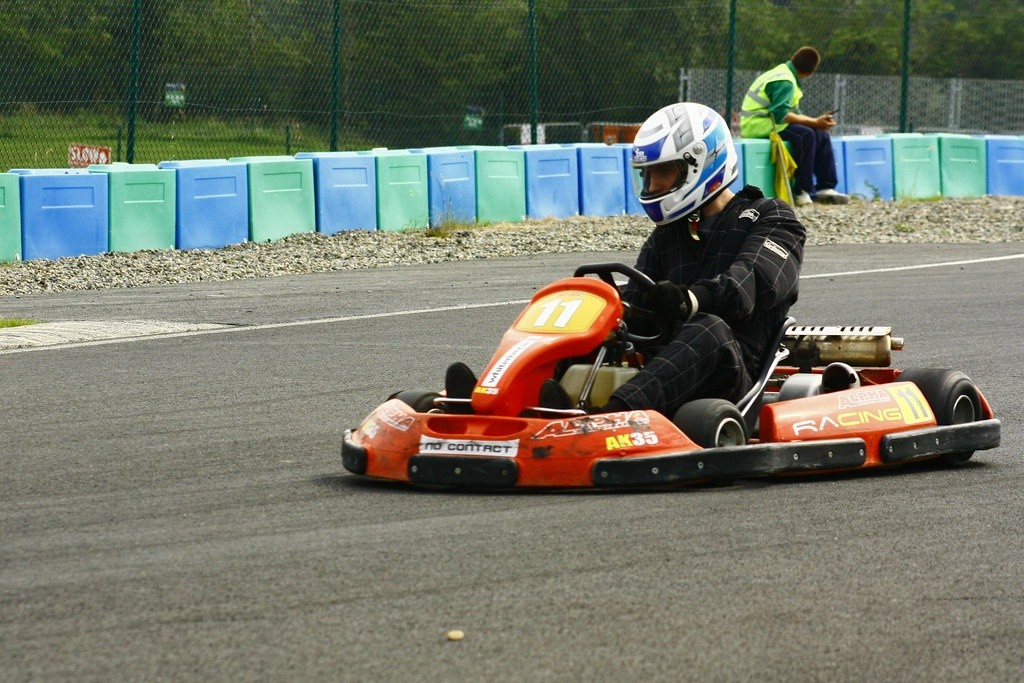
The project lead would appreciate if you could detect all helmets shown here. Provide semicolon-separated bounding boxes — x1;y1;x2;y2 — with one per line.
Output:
631;102;738;225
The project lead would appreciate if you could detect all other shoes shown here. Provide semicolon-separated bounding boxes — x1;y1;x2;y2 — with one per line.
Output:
539;378;574;419
796;192;813;206
445;362;477;414
816;189;849;204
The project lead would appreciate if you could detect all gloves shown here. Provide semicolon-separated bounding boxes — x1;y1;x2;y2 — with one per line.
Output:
648;280;692;322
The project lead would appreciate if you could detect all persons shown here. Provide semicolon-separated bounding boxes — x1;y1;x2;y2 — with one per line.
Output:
446;102;806;415
740;48;852;208
